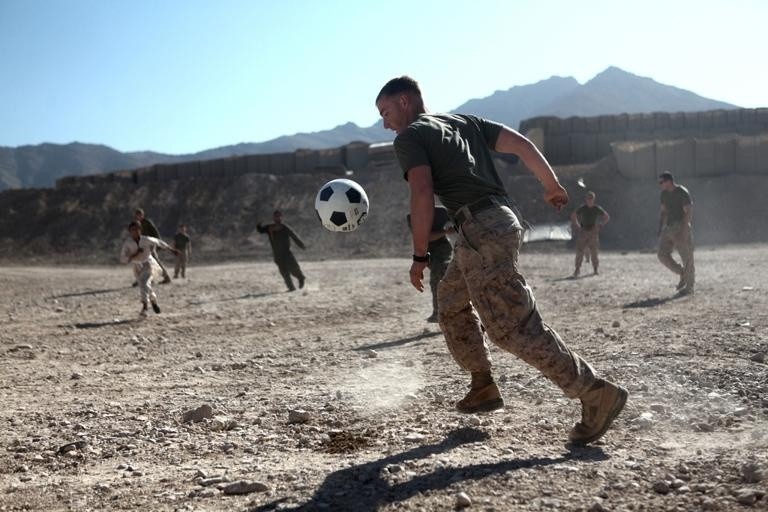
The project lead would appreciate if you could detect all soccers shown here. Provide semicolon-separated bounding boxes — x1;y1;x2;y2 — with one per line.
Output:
314;178;369;232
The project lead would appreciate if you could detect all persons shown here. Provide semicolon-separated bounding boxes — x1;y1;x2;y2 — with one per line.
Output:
172;225;193;279
130;207;172;287
657;169;695;295
121;219;183;318
407;206;457;324
257;209;308;294
375;75;629;447
568;191;612;277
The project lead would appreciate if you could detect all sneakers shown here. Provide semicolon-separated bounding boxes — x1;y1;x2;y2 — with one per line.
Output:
132;281;138;287
573;266;599;275
286;275;305;292
427;315;438;323
158;272;185;284
677;278;694;293
140;303;160;316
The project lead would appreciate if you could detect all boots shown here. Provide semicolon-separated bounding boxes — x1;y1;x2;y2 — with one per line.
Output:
569;378;629;442
455;371;504;412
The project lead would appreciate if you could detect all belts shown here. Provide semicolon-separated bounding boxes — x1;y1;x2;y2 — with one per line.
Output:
454;197;511;227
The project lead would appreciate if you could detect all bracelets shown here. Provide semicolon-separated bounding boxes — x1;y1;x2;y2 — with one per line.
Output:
412;251;431;264
